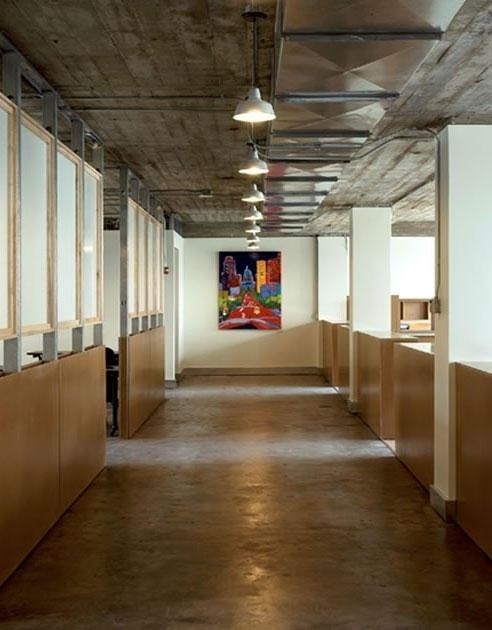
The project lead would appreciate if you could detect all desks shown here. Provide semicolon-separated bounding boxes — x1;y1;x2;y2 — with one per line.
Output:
27;352;62;360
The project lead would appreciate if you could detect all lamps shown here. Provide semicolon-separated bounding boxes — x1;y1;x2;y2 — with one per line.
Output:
232;11;277;250
